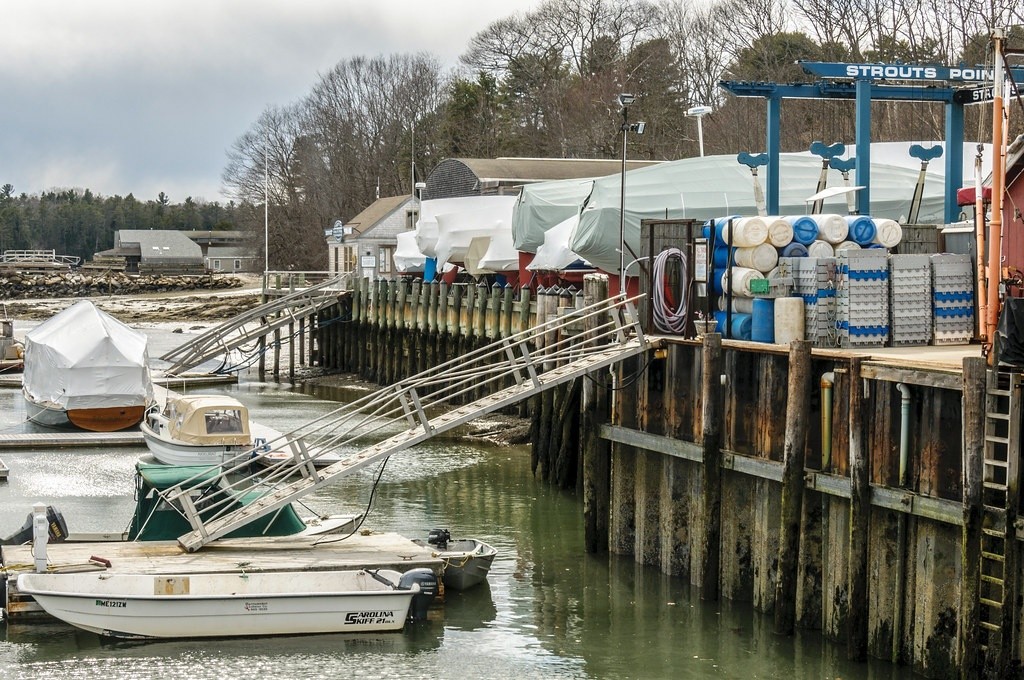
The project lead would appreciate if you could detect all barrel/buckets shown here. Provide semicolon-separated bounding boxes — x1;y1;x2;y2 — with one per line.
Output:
736;298;753;314
735;244;778;272
765;266;778;278
807;240;835;257
6;346;17;359
722;218;768;247
871;219;902;247
713;248;737;266
754;216;793;249
833;241;861;249
718;297;738;313
781;217;819;244
810;214;849;244
714;268;727;296
845;216;877;245
724;313;751;340
751;299;774;343
713;311;728;332
774;297;804;345
424;258;437;282
864;244;881;249
721;266;765;299
778;242;809;256
702;216;741;245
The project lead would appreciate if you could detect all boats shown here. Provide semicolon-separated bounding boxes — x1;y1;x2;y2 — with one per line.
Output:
139;371;270;474
392;141;1011;321
80;257;127;274
15;567;444;644
23;299;157;432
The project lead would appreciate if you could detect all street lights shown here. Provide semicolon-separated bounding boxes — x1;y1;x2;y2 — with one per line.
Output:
410;121;415;230
683;106;712;157
618;93;647;293
415;182;426;219
